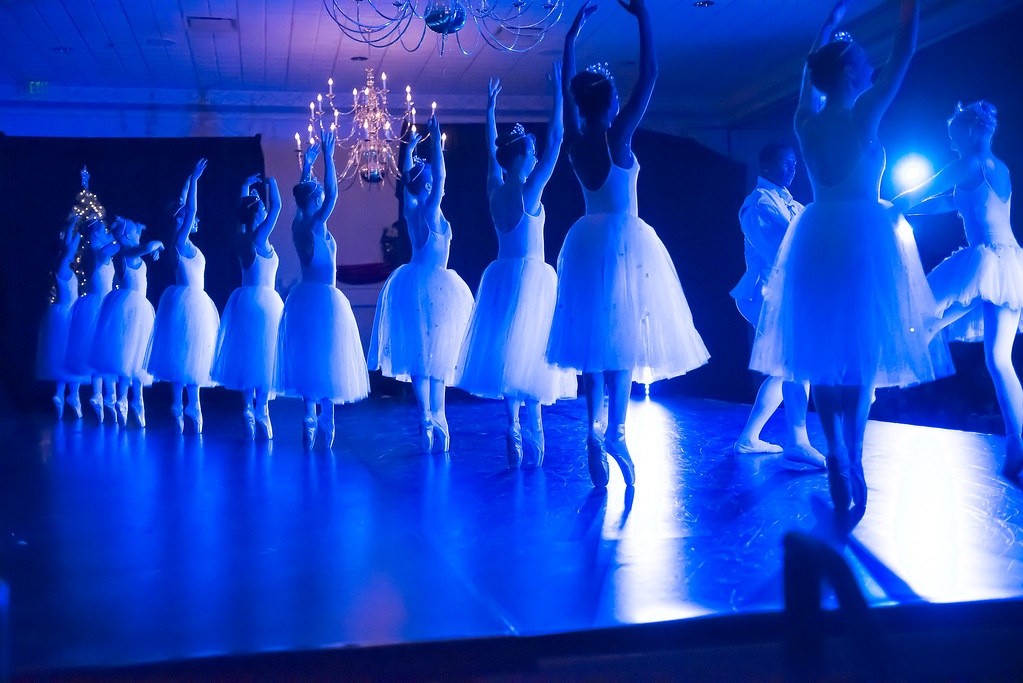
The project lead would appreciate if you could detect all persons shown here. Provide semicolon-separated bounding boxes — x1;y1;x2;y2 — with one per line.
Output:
457;59;577;468
892;101;1023;479
274;129;370;450
753;1;955;511
368;116;475;453
548;0;710;484
67;214;120;425
732;141;826;471
143;156;219;434
209;172;282;439
36;217;84;418
91;217;164;426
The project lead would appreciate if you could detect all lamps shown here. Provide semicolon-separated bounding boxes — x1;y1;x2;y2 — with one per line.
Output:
293;28;448;193
323;0;566;57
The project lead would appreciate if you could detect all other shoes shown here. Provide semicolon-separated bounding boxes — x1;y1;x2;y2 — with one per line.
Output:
587;420;609;487
170;404;184;434
302;413;317;453
90;393;104;423
255;408;273;440
116;396;129;426
185;404;202;433
67;394;82;418
420;411;449;454
735;439;783;453
53;392;64;419
604;424;635;486
243;408;255;442
129;397;145;428
789;447;828;469
506;417;544;468
317;412;335;449
105;395;118;422
1002;434;1023;477
826;440;867;509
